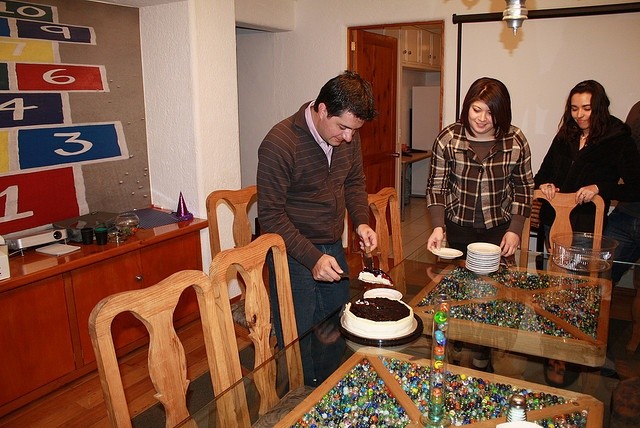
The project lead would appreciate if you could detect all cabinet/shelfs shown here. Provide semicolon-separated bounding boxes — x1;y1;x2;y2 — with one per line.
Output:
421;27;441;72
73;206;212;375
0;244;77;417
399;26;420;68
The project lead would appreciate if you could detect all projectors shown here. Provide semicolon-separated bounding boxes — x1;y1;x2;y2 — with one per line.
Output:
6;228;73;256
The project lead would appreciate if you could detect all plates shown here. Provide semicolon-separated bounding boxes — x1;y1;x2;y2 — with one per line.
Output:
431;246;464;260
363;288;403;301
465;243;501;275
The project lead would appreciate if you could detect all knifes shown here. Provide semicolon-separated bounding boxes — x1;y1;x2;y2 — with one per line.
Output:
336;272;358;282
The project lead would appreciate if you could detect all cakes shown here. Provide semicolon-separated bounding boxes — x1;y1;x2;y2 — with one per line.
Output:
358;266;397;287
340;296;415;340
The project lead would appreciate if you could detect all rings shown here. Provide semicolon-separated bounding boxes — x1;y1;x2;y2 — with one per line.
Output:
547;184;551;189
580;193;586;197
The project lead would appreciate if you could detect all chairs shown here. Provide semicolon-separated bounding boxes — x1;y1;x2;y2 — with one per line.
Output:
519;189;606;284
206;185;271;381
356;187;418;305
209;232;314;427
86;269;238;428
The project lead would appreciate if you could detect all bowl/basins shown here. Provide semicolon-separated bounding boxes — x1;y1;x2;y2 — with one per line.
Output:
553;231;619;273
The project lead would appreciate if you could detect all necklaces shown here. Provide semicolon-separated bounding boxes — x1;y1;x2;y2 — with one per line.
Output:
580;135;587;140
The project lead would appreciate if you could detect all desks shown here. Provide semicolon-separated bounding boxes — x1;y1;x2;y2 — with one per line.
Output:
401;150;432;221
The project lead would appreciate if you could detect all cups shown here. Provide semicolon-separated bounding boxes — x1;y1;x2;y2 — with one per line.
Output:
80;228;93;245
94;227;107;245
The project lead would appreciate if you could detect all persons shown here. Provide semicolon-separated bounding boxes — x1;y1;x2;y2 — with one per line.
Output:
424;77;535;269
255;68;378;388
542;357;580;388
605;262;640;424
604;100;640;264
313;319;341;346
532;80;627;252
